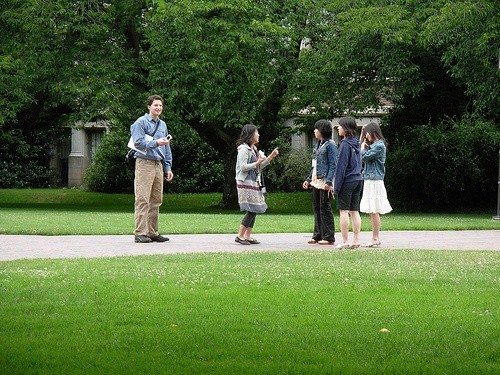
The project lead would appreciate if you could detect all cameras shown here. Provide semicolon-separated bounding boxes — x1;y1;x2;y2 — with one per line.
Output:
164;134;173;142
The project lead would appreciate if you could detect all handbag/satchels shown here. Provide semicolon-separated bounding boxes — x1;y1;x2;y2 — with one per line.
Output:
125;149;136;170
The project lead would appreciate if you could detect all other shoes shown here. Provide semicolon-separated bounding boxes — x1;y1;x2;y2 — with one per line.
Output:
150;235;169;242
248;238;260;244
135;235;153;243
318;240;334;245
235;236;251;245
350;242;361;249
307;239;317;244
334;241;350;249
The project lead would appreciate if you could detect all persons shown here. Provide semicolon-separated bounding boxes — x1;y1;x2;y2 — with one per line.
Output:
332;116;365;250
359;122;392;248
235;124;279;245
303;119;339;246
130;95;174;243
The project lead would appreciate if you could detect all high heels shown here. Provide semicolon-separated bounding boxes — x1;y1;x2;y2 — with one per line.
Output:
378;241;381;247
365;241;378;247
